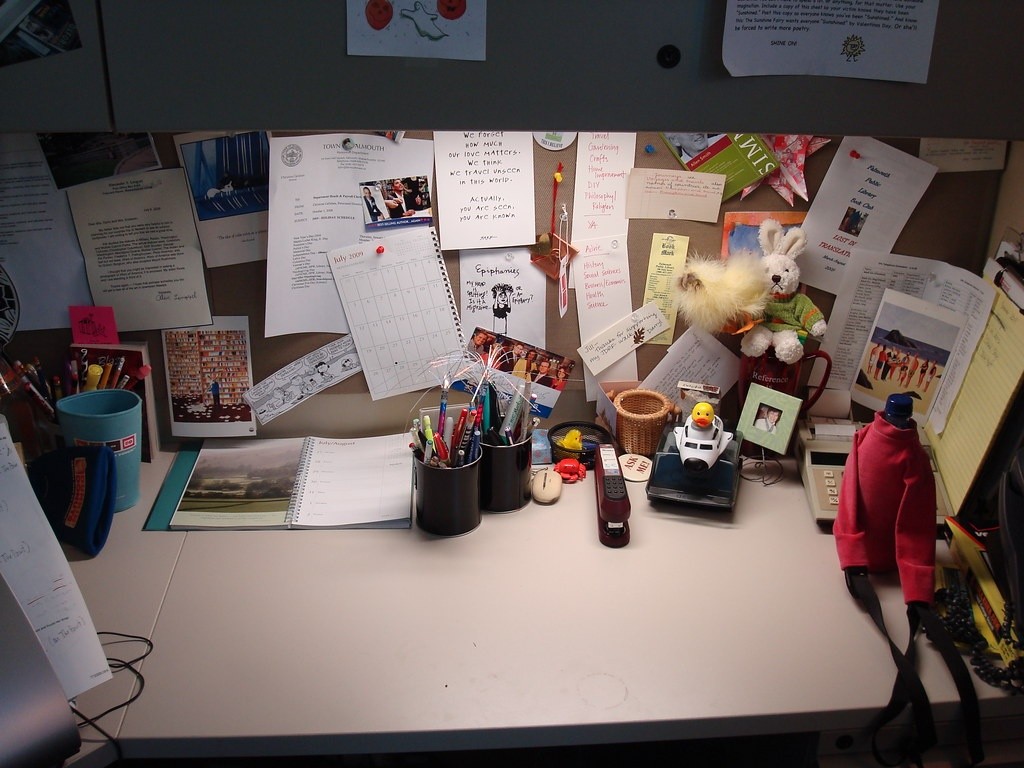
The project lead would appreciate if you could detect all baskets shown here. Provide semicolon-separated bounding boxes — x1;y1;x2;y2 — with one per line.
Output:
613;389;672;457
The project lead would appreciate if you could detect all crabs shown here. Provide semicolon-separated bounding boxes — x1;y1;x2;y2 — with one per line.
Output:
554;458;586;484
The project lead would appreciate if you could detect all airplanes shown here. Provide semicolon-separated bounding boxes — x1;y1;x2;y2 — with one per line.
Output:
672;416;734;476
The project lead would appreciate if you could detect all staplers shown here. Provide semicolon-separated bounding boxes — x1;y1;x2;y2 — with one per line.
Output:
593;443;632;548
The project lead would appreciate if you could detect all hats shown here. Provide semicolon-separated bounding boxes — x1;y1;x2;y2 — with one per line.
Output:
27;446;117;557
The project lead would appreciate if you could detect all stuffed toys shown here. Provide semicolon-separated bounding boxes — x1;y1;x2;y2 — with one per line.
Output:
733;218;827;363
558;428;582;460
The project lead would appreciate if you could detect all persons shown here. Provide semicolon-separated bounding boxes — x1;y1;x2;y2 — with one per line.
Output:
865;341;938;393
360;177;428;223
752;403;782;434
462;328;568;390
209;379;221;407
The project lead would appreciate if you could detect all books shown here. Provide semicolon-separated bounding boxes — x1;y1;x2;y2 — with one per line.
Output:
169;433;413;531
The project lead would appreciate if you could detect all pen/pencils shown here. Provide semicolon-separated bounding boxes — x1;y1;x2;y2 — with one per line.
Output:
0;347;152;426
480;380;541;446
408;384;486;468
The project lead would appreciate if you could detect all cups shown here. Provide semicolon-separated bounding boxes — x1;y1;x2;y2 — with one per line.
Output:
479;434;532;515
56;390;142;513
414;445;484;536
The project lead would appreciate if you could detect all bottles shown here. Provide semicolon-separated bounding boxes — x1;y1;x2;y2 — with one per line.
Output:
884;394;913;429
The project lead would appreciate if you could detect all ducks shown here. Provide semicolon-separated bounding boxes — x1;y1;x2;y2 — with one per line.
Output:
673;402;733;475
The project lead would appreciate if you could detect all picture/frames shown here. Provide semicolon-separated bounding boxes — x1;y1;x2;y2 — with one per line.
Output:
736;382;803;456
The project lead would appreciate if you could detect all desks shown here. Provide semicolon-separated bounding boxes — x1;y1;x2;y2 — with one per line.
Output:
59;449;1024;768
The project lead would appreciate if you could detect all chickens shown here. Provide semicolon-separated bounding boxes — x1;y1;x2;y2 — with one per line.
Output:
557;430;583;459
